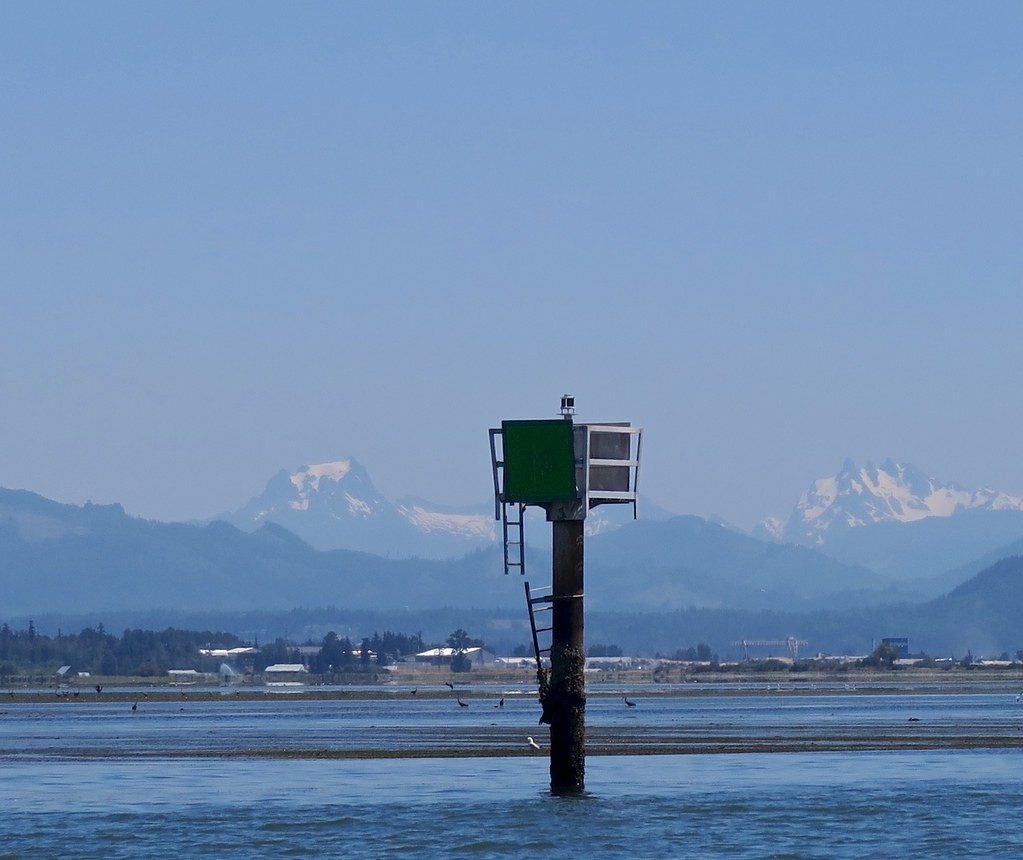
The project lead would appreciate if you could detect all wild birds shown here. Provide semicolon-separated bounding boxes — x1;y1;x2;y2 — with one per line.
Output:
528;737;542;749
8;684;270;699
622;695;636;707
132;699;139;710
411;682;504;708
